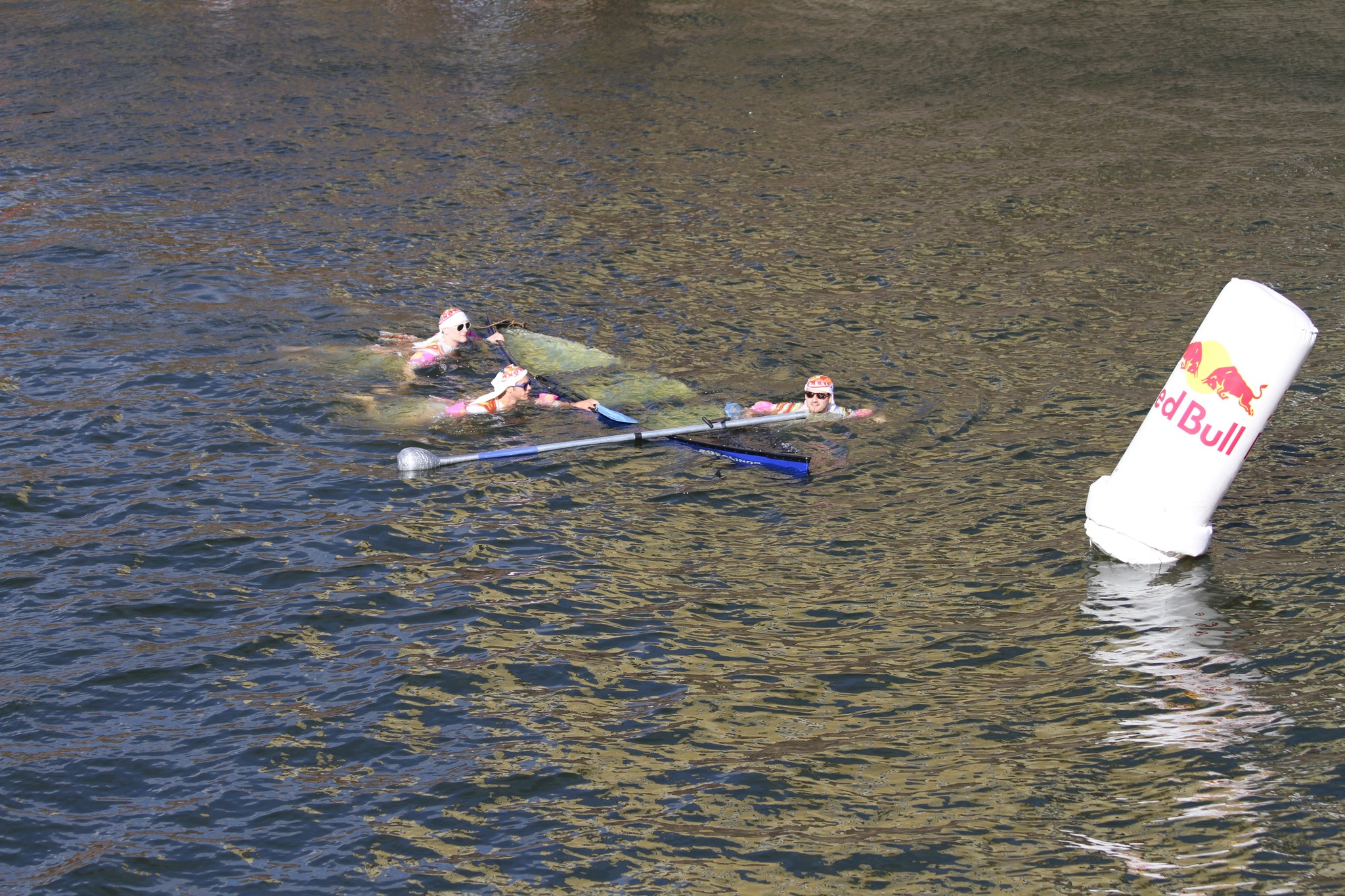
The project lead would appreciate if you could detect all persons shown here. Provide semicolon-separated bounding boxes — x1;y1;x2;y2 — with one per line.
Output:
372;308;505;381
435;363;600;418
723;375;872;423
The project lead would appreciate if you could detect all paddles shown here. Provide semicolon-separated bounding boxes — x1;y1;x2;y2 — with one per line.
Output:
535;374;639;423
702;401;741;429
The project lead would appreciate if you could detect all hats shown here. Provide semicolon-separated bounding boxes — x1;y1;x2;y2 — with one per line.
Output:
438;309;468;329
491;363;527;393
805;374;834;393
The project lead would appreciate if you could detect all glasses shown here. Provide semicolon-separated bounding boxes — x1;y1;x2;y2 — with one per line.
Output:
453;321;471;331
805;391;828;400
516;379;532;390
